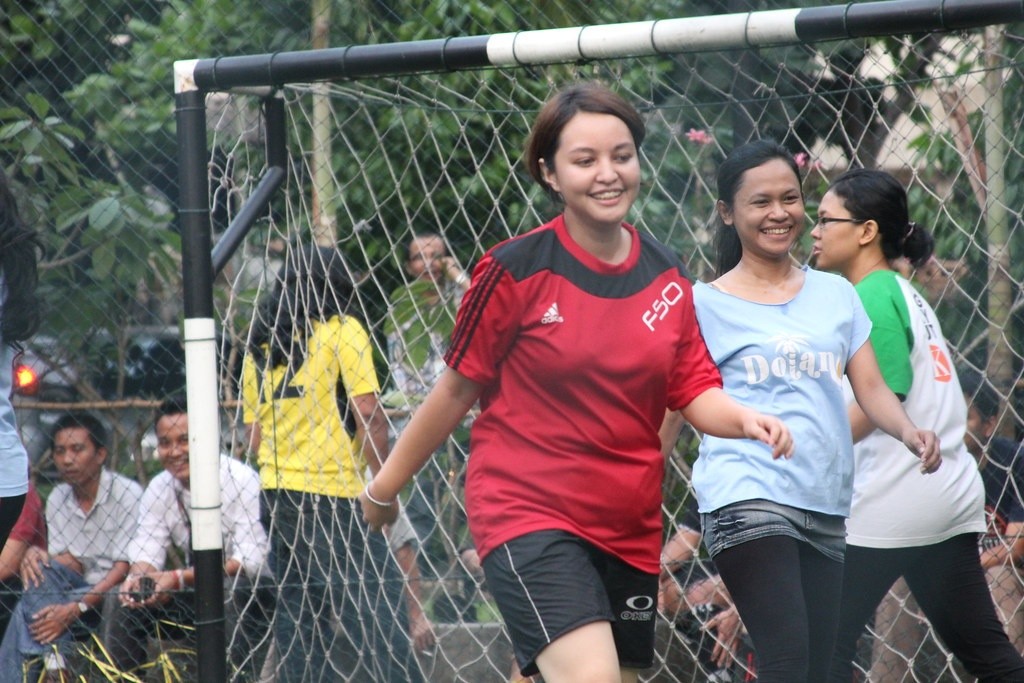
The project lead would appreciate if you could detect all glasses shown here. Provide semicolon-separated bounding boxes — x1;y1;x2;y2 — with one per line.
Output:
814;217;867;227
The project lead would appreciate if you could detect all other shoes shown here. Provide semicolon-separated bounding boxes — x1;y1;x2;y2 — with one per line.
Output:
44;651;65;671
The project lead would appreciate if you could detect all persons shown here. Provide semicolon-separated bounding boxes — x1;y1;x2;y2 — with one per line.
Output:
357;85;795;683
811;167;1024;683
240;246;422;683
657;141;943;683
255;377;435;683
106;394;274;683
381;230;489;622
0;413;145;683
658;366;1024;683
0;168;47;631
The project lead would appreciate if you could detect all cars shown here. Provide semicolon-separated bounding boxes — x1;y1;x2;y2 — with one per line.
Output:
16;326;252;418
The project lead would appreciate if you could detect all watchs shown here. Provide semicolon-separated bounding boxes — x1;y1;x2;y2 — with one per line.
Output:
77;600;87;613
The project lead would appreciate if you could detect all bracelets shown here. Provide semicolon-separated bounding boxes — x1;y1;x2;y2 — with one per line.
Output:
365;485;394;508
171;569;185;592
456;272;467;284
